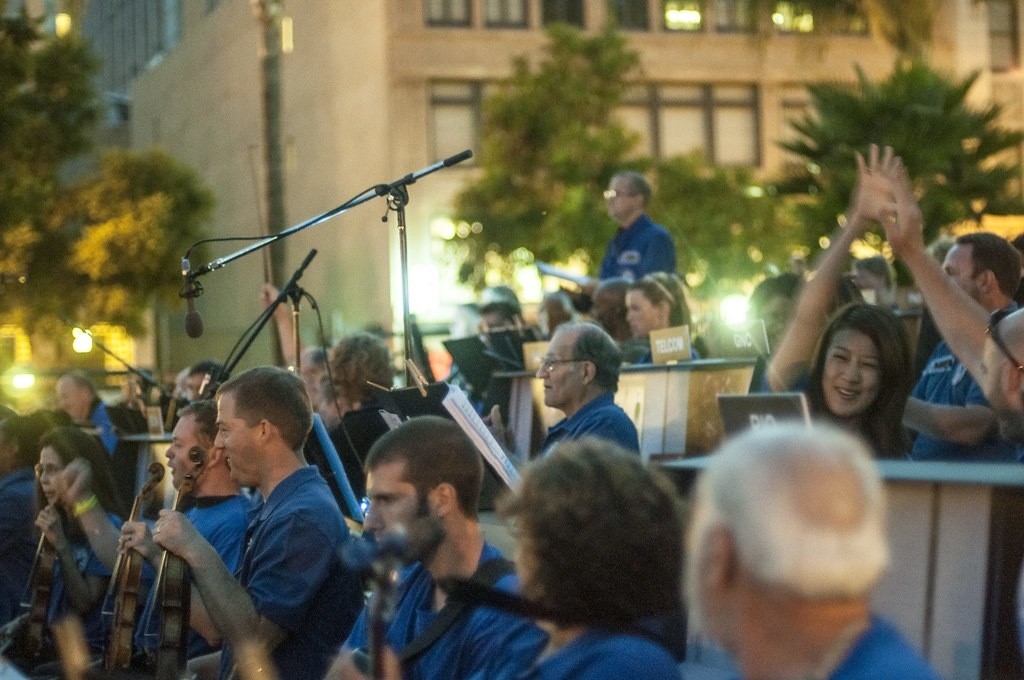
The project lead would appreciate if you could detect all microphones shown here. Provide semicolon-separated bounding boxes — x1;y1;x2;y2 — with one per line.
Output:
182;258;204;339
199;366;216;397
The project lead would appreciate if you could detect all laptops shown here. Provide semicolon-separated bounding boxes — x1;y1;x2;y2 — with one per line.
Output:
716;392;811;440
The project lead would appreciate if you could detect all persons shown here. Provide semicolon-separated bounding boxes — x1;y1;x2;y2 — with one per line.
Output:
573;169;677;296
448;271;708;419
493;436;687;680
686;421;949;680
480;321;642;483
323;414;551;680
744;142;1024;466
259;281;407;522
0;360;366;680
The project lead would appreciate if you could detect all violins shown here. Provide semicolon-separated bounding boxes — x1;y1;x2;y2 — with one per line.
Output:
20;487;64;670
95;461;167;680
151;444;212;680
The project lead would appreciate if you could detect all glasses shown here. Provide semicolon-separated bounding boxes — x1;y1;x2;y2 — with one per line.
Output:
985;302;1024;374
603;189;628;201
538;356;586;372
34;462;66;476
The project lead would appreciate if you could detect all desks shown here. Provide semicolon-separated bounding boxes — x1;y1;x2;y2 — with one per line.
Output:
529;358;753;472
650;455;1022;678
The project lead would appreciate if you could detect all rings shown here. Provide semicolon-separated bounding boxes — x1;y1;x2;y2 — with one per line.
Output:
156;526;161;532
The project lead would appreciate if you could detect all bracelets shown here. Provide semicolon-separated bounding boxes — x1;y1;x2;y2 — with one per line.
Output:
73;496;97;518
